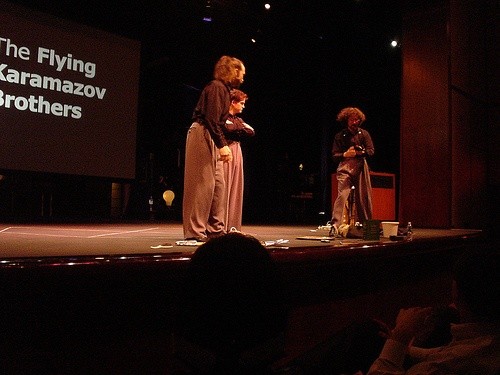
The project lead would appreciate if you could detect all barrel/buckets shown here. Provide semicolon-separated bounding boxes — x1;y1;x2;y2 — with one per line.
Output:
382;222;399;237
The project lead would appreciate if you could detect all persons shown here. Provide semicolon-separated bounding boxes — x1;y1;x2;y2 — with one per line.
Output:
330;107;376;228
183;55;255;243
361;259;499;374
222;90;253;235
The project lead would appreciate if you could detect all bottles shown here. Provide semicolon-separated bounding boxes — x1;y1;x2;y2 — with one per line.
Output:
407;222;413;240
